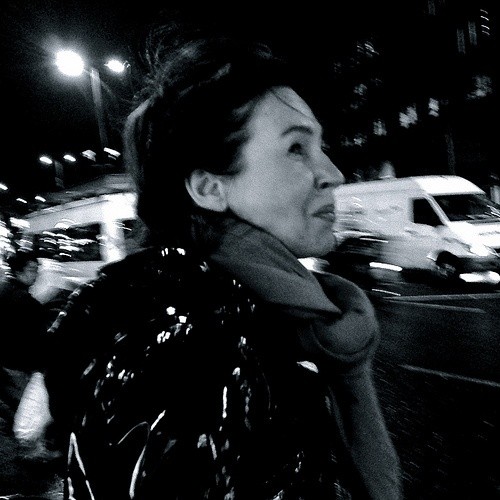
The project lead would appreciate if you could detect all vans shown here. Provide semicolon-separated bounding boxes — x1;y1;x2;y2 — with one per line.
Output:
17;191;146;293
333;174;500;288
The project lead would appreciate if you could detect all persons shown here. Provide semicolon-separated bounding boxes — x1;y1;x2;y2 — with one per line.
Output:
3;249;72;404
13;34;419;500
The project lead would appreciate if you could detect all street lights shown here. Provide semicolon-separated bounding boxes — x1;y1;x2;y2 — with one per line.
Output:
59;50;107;147
